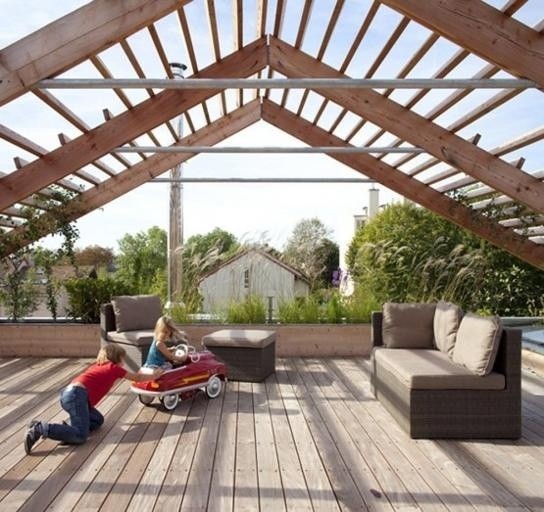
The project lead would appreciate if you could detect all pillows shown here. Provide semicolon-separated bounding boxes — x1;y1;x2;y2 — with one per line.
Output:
108;294;163;332
378;300;503;376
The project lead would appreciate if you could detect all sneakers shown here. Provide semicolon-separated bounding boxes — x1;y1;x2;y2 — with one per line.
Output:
24;420;42;455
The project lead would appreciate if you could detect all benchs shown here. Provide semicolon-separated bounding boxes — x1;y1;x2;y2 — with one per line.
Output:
369;299;523;442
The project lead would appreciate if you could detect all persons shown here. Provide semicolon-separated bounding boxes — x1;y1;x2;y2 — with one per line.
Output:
145;315;183;369
22;343;163;456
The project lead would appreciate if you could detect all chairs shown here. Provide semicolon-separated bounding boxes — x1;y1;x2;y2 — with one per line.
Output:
98;303;185;375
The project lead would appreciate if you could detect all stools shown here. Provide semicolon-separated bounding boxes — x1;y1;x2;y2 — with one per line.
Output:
201;327;277;384
